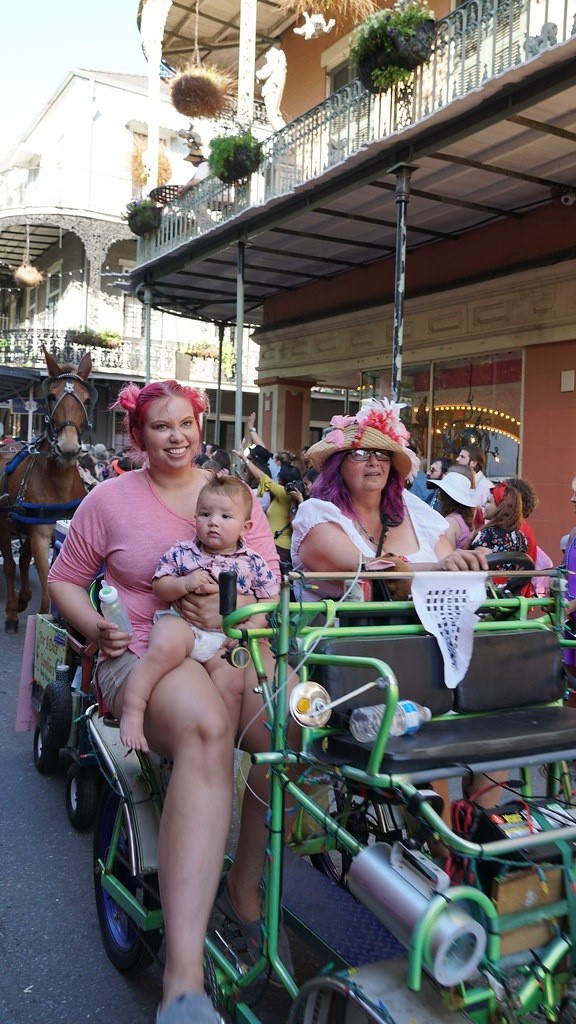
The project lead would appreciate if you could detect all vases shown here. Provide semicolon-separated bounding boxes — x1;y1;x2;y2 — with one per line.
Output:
355;20;436;96
176;352;215;381
127;207;161;235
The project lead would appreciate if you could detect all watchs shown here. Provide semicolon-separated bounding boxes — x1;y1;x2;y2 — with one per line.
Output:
249;428;256;432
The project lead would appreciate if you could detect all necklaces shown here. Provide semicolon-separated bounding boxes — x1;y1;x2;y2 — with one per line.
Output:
355;516;389;545
203;542;239;555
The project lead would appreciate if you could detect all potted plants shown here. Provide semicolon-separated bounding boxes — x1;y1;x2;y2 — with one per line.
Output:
206;125;263;185
69;329;120;349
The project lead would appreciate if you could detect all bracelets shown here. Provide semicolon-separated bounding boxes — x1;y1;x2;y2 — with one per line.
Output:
245;460;250;463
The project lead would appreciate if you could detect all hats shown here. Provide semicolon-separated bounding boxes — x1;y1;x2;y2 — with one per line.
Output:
122;445;132;449
112;459;132;475
248;445;274;470
92;443;106;454
81;444;88;452
107;448;115;450
303;397;420;484
428;471;482;507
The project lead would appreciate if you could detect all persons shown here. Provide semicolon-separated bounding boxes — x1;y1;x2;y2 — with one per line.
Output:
47;381;300;1024
290;403;509;858
405;441;575;676
120;476;280;752
75;443;145;492
193;412;319;571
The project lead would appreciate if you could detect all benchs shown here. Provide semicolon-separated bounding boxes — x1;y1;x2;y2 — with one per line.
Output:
297;619;575;784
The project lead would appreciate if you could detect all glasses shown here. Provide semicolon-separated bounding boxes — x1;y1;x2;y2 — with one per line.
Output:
347;449;393;461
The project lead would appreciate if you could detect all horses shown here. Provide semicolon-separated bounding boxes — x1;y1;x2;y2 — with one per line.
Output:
0;343;98;635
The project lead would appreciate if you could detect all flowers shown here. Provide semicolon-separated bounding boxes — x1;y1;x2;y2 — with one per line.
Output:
341;0;438;68
176;340;212;363
120;197;156;223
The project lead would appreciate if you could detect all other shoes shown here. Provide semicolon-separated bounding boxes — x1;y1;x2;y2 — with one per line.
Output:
214;871;296;988
157;993;224;1023
